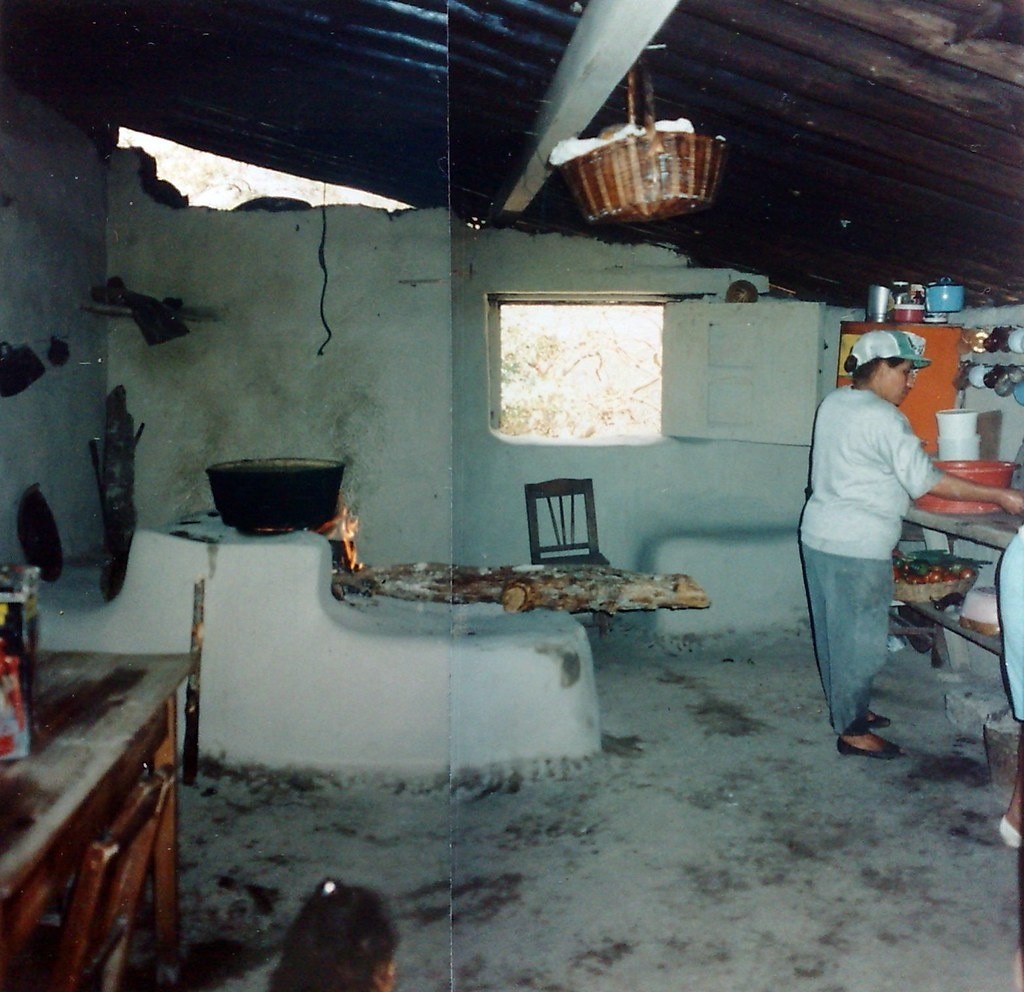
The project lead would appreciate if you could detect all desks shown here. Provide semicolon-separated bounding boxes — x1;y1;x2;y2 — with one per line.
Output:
1;650;198;992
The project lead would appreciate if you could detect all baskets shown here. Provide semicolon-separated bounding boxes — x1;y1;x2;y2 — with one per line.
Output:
560;57;727;223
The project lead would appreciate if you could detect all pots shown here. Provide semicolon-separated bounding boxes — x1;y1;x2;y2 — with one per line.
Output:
17;483;65;585
204;456;346;532
922;276;966;313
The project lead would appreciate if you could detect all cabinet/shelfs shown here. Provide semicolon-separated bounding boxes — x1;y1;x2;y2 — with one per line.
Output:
899;496;1024;657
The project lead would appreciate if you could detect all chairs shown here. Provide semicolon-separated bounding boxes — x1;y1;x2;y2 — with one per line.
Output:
2;762;178;992
524;479;610;640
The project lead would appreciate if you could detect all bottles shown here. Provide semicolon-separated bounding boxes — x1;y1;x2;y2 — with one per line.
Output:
892;280;925;305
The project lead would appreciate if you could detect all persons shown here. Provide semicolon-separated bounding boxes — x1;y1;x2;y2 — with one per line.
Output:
997;525;1024;850
269;877;399;992
800;329;1024;761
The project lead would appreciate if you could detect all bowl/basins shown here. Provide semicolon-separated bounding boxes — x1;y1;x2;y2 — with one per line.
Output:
960;585;998;625
913;458;1021;514
892;305;925;323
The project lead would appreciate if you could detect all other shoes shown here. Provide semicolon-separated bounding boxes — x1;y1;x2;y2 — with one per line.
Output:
998;815;1024;848
838;733;905;758
866;710;891;729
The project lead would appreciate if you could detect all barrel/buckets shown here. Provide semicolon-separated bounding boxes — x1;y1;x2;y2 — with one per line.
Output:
934;408;982;460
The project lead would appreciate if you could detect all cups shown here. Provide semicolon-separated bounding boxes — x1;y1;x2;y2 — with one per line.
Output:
866;278;890;323
952;327;1024;397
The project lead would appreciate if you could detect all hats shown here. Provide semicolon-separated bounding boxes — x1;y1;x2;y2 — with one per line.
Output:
849;327;931;377
